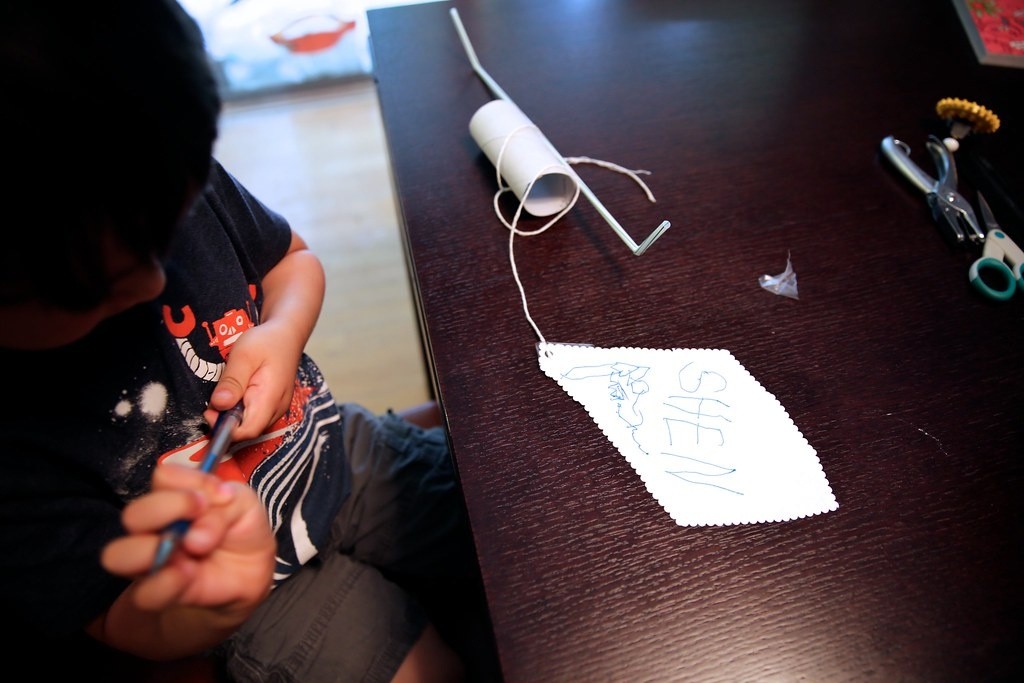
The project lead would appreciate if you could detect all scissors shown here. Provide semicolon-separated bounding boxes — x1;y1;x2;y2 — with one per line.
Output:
964;189;1024;304
879;132;985;244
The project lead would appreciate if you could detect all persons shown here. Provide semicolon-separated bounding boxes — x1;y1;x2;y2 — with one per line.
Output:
1;1;497;683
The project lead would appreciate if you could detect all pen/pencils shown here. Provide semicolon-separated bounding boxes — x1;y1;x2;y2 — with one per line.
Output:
148;397;246;577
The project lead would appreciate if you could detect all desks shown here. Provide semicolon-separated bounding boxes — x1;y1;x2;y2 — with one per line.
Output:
364;0;1024;683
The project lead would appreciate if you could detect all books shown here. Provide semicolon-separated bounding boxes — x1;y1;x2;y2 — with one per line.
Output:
952;0;1024;69
